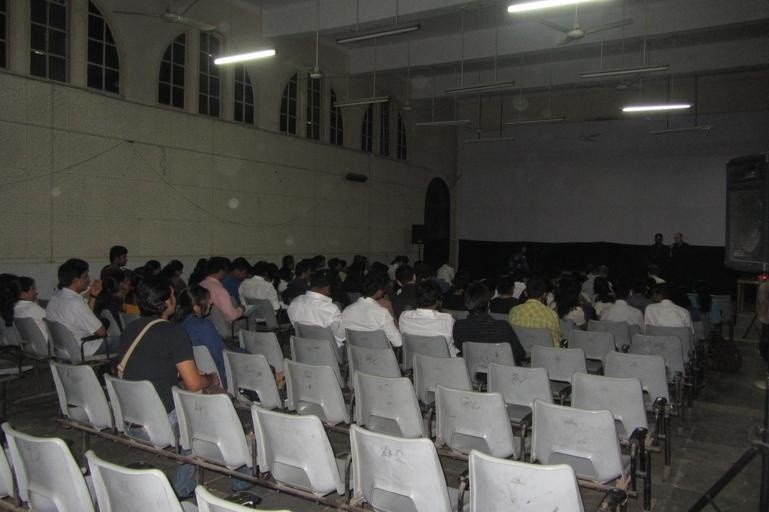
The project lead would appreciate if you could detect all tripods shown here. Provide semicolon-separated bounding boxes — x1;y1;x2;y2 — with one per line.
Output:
688;377;769;511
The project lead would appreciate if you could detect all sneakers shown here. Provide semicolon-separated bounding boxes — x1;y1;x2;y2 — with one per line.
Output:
754;378;769;390
232;471;271;491
176;490;194;500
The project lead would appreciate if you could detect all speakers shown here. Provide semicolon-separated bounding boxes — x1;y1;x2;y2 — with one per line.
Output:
723;151;769;274
412;225;427;244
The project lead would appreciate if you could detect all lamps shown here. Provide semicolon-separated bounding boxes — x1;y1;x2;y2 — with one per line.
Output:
619;77;692;112
578;2;670;78
415;65;471;126
213;3;276;65
504;85;565;125
333;40;391;107
337;1;421;45
444;9;515;94
649;77;711;135
509;0;577;14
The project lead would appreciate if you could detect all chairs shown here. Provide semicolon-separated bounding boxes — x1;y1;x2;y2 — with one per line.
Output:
1;261;713;511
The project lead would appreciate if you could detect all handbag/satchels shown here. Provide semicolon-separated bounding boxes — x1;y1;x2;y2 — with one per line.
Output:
707;335;743;372
